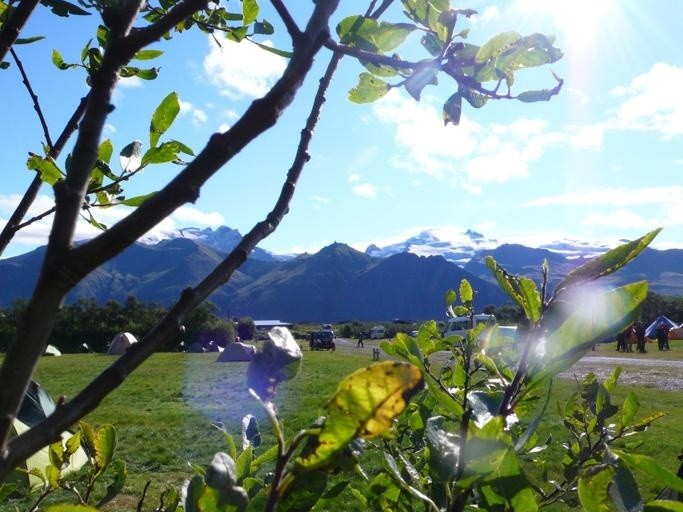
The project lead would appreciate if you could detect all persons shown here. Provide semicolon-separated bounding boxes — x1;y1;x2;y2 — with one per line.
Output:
175;321;185;352
661;323;669;350
614;321;646;353
79;341;88;353
356;335;363;349
654;324;663;350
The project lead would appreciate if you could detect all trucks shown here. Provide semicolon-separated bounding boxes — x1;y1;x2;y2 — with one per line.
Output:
443;314;520;344
321;323;331;330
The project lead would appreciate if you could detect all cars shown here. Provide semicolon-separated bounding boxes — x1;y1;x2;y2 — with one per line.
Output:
410;330;418;337
358;325;395;339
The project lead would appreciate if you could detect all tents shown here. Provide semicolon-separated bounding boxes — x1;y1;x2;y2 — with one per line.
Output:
214;341;256;361
668;323;682;340
106;332;138;355
644;315;678;339
43;344;61;357
629;328;650;343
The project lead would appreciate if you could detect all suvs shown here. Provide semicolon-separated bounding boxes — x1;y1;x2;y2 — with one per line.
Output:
309;330;335;352
